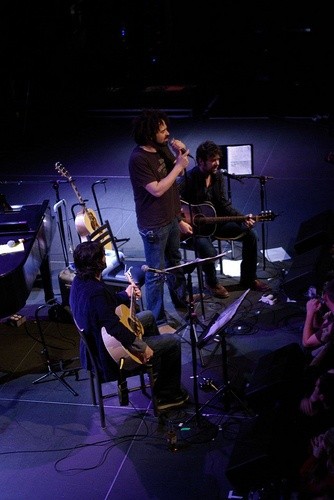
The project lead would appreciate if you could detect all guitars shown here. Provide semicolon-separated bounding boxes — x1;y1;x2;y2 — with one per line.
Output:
101;271;149;371
53;161;115;251
179;203;277;238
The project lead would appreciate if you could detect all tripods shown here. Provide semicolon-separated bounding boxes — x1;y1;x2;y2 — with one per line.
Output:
30;297;79;396
182;291;258;425
158;250;225;367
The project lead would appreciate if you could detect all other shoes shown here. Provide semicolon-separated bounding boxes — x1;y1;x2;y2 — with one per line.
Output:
151;388;189;410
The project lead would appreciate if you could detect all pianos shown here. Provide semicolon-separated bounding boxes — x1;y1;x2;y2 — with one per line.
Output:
0;199;57;320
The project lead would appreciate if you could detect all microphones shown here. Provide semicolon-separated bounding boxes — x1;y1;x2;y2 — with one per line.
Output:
170;140;193;158
141;264;173;275
219;169;240;182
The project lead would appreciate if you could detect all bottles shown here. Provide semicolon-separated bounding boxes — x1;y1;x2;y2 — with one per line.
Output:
167;419;178;453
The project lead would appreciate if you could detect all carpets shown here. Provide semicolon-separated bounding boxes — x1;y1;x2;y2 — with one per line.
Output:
0;320;83;373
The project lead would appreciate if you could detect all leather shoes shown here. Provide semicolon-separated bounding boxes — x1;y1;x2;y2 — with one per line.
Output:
240;278;268;290
211;283;230;298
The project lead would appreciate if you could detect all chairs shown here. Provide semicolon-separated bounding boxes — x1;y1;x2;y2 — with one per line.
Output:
73;316;157;428
179;211;223;289
88;220;149;310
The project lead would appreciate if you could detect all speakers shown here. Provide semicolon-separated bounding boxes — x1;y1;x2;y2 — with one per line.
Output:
280;245;334;297
293;208;334;256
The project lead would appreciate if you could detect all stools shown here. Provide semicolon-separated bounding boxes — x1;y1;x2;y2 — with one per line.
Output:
58;266;78;306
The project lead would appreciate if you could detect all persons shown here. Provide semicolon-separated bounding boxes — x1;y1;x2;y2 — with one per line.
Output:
129;108;207;337
262;279;334;500
180;141;269;298
69;242;190;409
